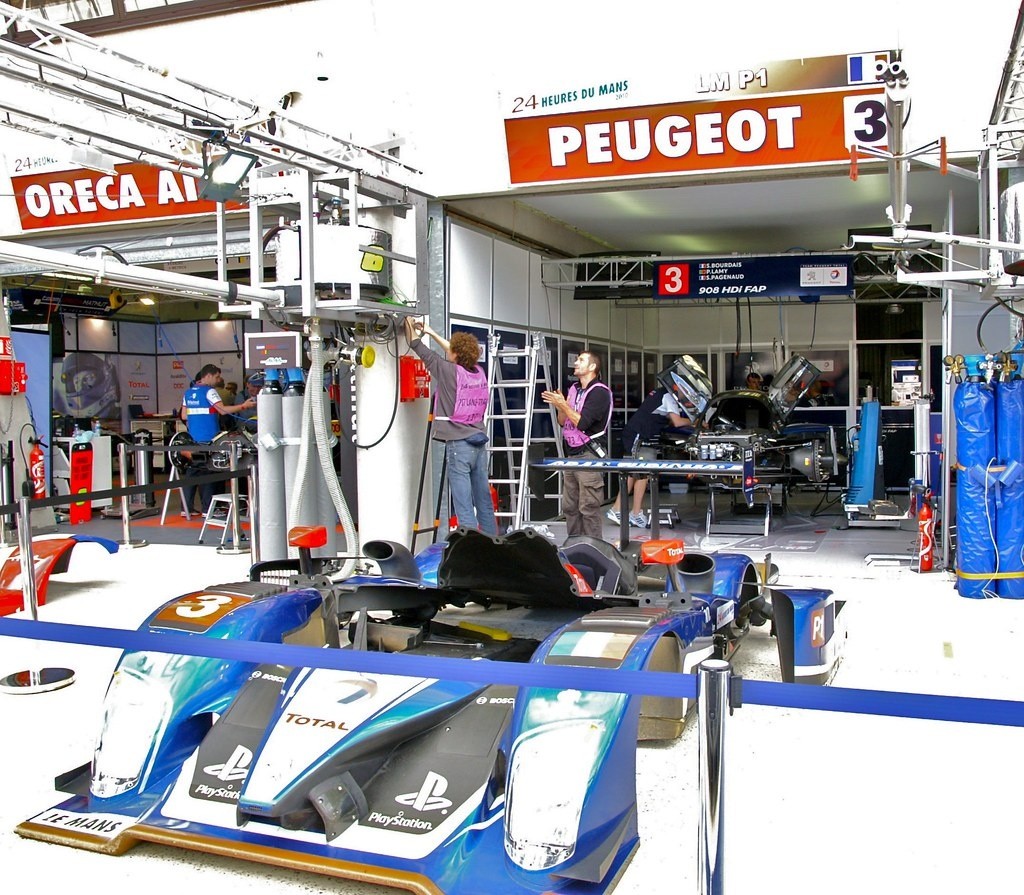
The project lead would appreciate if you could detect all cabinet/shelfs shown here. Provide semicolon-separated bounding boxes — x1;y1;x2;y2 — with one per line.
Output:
130;420;172;470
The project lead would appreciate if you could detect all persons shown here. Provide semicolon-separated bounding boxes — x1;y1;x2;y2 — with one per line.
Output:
542;351;613;541
606;375;709;528
405;316;498;536
61;353;122;419
745;373;825;408
180;364;264;518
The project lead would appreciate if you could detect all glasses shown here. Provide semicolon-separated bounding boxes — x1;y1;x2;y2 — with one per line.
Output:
225;387;234;390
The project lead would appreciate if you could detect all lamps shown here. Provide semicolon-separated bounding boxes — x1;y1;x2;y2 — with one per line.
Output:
196;139;258;204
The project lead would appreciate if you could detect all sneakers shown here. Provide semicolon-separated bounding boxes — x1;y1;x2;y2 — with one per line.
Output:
628;508;649;528
605;509;620;525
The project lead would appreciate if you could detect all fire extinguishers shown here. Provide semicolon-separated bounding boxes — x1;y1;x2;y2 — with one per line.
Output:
917;486;941;573
20;423;48;507
478;474;499;535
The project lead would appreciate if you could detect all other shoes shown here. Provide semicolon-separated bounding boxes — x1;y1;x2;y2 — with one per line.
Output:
203;508;226;517
181;508;200;516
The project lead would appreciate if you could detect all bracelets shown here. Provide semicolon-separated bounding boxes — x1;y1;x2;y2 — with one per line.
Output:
241;405;245;410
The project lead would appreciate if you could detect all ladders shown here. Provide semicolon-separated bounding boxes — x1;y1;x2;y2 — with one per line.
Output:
482;331;567;532
